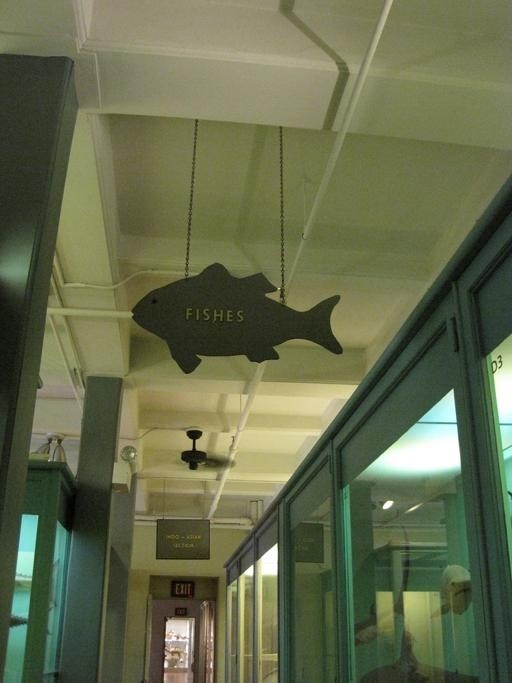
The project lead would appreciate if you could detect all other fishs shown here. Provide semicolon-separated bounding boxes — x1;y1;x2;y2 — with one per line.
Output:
430;563;474;618
